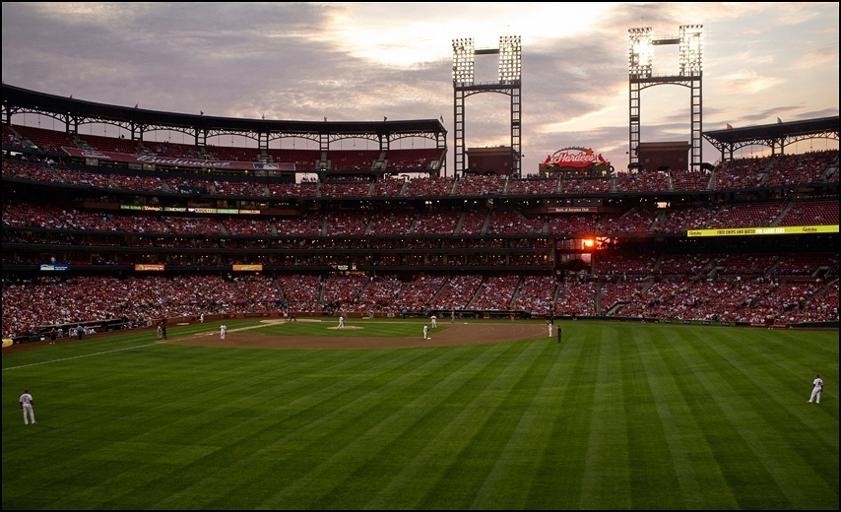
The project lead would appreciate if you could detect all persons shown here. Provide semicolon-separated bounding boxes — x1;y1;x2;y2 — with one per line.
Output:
217;323;227;341
334;147;840;337
156;317;166;340
557;325;562;343
49;328;58;344
805;373;824;404
18;387;36;426
76;323;83;339
156;321;162;340
422;324;428;340
1;122;348;336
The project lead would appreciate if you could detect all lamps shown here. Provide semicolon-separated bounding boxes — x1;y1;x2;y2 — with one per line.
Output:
452;24;703;85
